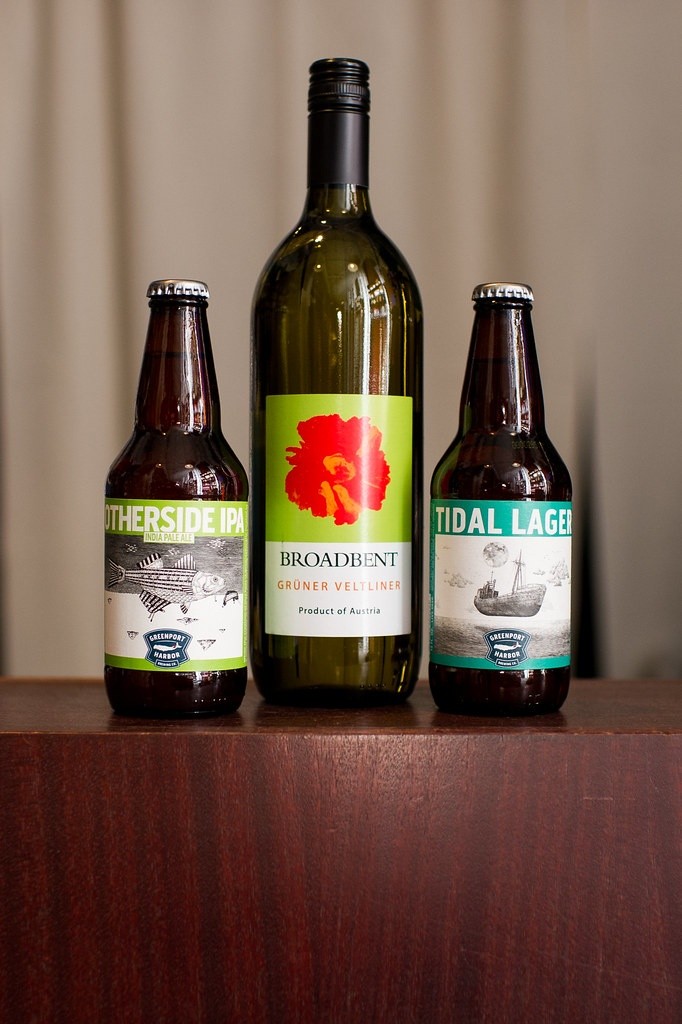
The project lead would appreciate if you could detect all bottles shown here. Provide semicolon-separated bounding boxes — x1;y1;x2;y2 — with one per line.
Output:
104;278;252;720
251;57;425;711
428;281;574;718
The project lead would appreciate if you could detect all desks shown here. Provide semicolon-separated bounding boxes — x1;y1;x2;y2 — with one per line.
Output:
0;680;682;1024
